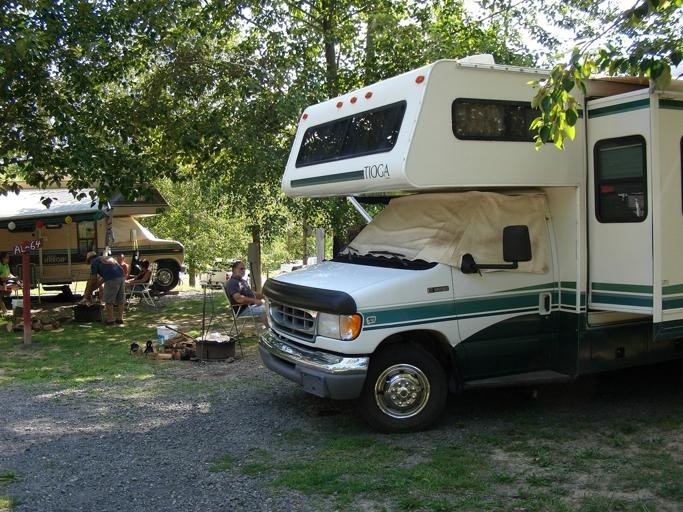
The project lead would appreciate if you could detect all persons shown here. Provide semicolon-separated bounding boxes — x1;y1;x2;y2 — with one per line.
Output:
0;251;15;315
84;250;126;325
225;259;268;328
126;259;152;293
117;253;130;277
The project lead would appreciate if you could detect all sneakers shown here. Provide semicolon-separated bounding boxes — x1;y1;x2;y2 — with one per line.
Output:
104;318;123;325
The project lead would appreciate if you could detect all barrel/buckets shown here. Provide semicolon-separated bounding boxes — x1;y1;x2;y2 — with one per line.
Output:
157;325;177;346
193;336;236;360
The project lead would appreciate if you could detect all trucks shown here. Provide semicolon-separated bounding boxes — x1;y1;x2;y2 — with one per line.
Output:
256;52;683;434
0;172;184;291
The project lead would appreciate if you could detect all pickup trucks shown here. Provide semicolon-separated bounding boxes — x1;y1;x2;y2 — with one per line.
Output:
198;259;250;290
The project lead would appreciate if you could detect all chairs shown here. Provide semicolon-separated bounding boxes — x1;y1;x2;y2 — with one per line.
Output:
218;283;265;355
14;263;42;306
125;270;159;314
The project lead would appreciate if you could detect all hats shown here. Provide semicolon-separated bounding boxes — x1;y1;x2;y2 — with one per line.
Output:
85;251;98;265
